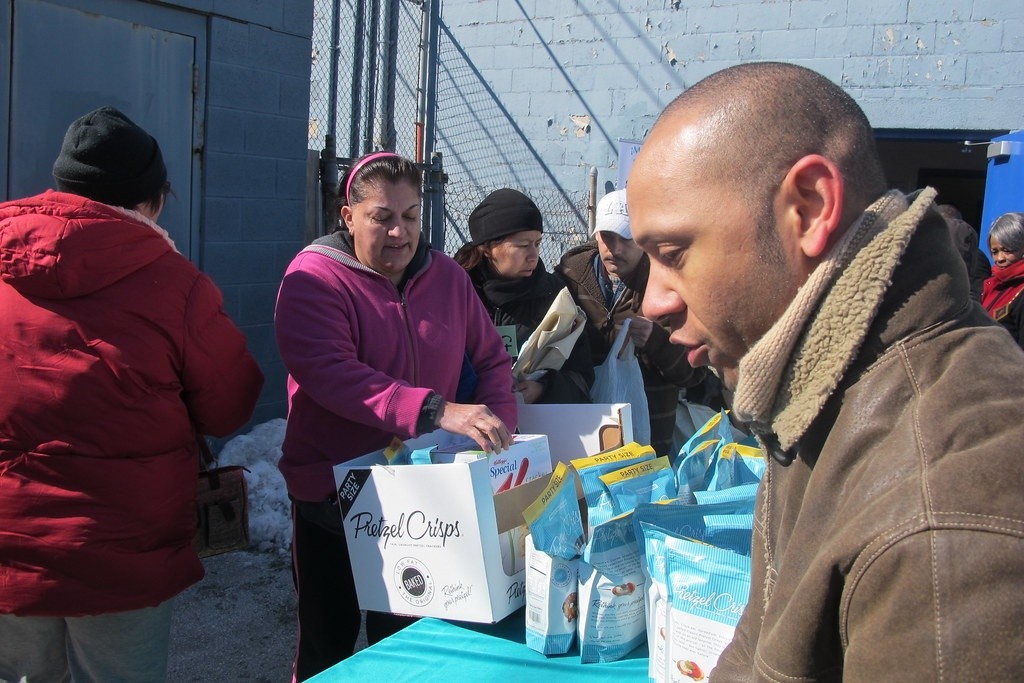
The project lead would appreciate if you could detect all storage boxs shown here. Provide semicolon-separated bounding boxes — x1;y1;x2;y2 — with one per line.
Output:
332;399;634;623
408;433;554;574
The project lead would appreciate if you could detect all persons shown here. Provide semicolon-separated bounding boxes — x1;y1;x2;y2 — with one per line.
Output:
452;186;586;405
274;149;521;683
0;106;265;683
628;59;1024;683
981;213;1024;341
551;191;691;461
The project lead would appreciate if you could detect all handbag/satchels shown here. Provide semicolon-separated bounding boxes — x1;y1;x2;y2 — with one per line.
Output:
187;463;254;558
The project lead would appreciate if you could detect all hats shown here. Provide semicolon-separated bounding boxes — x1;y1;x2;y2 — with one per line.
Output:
588;188;633;240
51;104;171;204
468;187;543;246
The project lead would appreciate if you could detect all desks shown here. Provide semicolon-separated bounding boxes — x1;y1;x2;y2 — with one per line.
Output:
303;606;656;683
